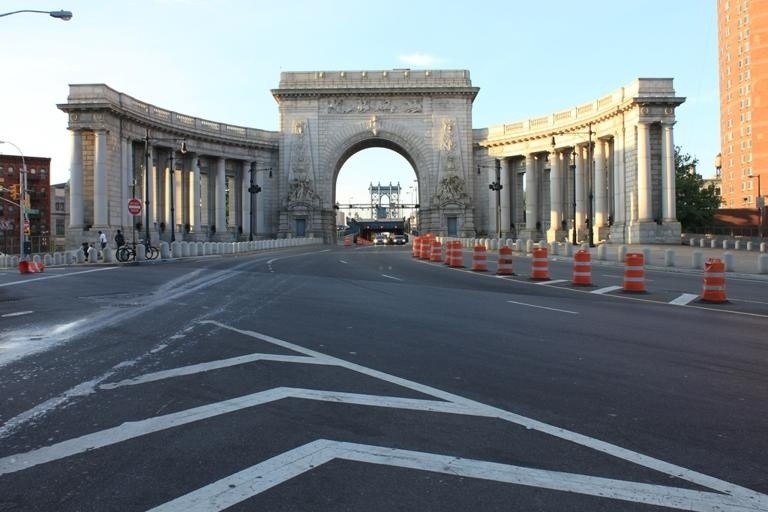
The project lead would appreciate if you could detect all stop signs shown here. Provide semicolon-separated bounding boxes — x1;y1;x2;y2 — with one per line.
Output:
128;199;141;214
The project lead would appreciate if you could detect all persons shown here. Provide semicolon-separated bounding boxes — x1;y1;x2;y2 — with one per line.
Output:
98;231;107;252
115;230;124;248
80;242;93;261
134;223;144;244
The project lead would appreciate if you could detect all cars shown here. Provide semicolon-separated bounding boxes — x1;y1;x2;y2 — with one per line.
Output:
373;233;407;245
337;224;345;231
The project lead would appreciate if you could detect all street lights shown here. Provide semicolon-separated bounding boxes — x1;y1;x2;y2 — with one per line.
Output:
1;10;72;21
551;132;594;247
477;165;501;238
406;179;419;229
748;176;761;225
249;167;272;241
142;137;186;239
0;140;28;259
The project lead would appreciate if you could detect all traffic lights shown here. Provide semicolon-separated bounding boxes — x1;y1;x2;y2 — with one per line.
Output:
9;184;17;201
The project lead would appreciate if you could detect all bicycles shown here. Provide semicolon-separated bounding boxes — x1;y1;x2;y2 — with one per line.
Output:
116;240;159;262
